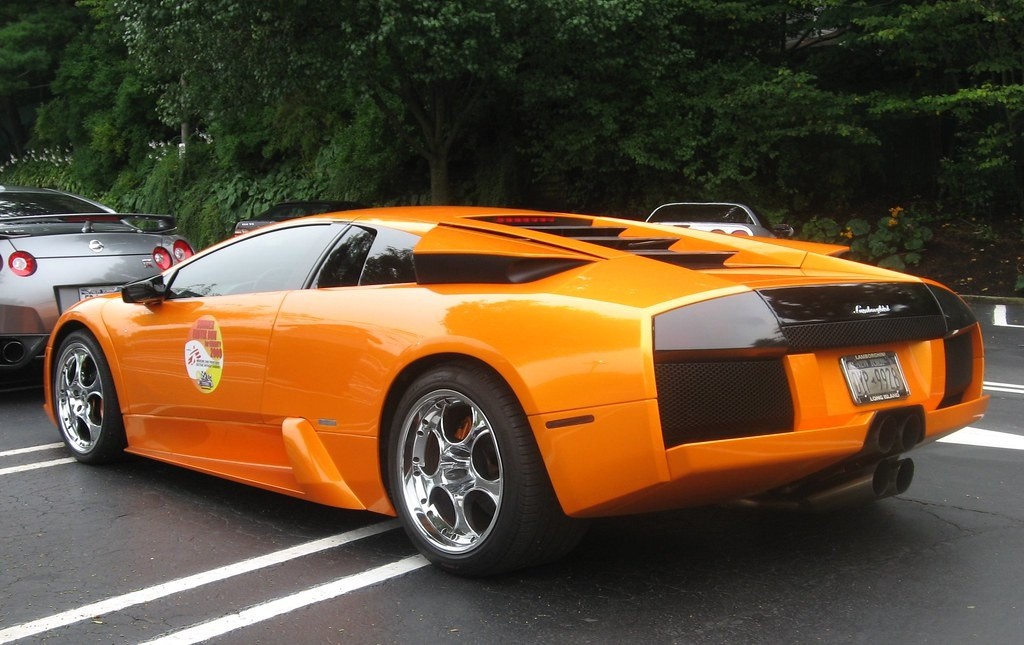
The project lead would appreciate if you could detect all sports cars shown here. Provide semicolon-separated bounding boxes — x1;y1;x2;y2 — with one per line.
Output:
40;201;991;581
0;182;197;394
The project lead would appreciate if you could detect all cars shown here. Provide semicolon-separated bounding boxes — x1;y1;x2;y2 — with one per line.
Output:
642;201;795;239
231;198;373;238
